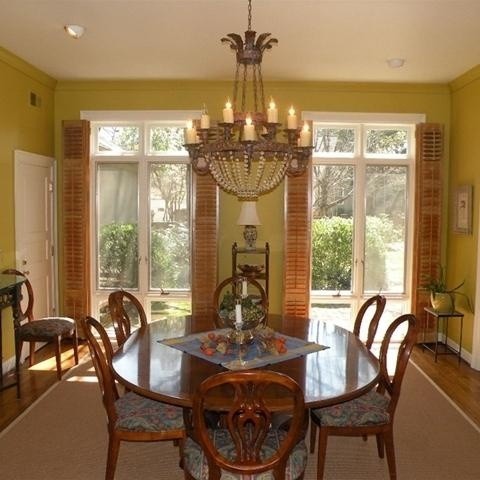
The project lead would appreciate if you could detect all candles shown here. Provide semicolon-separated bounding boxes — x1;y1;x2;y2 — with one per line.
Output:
241;277;248;297
233;299;243;326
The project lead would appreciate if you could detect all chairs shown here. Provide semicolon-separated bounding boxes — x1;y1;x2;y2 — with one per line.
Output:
108;290;148;348
82;316;190;480
350;292;387;351
310;313;419;480
180;369;308;480
1;269;79;381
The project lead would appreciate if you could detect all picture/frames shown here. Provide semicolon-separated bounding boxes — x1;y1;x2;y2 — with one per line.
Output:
452;184;474;235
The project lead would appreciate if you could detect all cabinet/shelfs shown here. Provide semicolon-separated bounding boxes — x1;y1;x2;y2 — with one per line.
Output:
231;242;270;304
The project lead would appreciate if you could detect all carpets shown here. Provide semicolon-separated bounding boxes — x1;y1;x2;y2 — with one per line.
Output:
0;337;480;479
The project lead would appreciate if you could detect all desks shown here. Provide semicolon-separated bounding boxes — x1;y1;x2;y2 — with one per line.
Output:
0;273;28;399
421;305;465;363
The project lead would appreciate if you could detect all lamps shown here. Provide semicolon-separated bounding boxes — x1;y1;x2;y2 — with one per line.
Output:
181;0;316;226
386;59;405;68
65;25;87;40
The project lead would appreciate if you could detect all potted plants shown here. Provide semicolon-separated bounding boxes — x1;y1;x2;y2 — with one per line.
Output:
418;262;472;314
